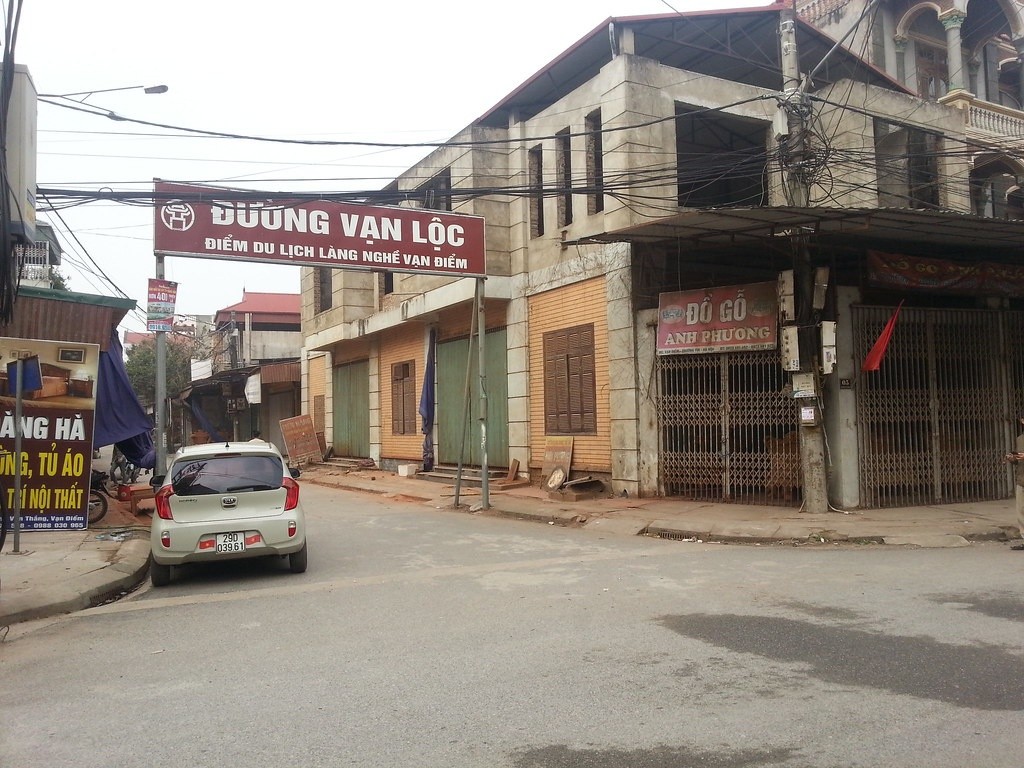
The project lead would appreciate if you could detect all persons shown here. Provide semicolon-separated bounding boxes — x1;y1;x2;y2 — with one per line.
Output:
247;431;265;443
122;453;142;483
110;442;128;487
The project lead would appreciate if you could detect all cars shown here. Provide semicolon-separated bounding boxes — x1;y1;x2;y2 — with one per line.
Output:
148;440;307;589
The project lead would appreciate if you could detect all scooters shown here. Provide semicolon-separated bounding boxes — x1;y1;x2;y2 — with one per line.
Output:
122;458;143;483
88;469;113;525
